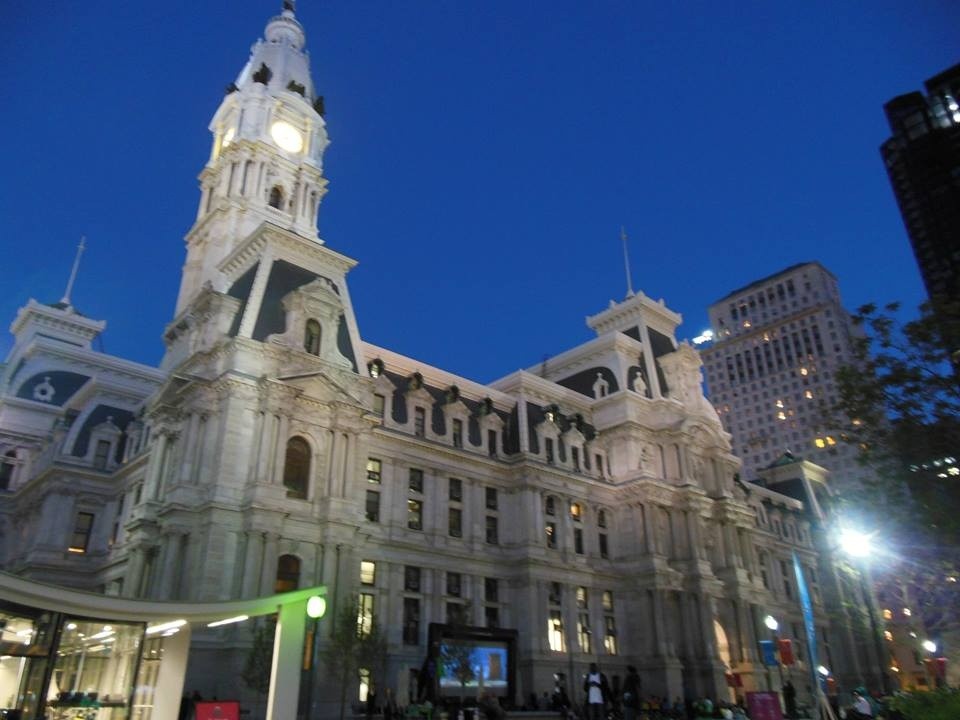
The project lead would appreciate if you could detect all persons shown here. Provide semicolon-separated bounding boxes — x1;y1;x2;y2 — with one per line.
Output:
647;695;688;720
622;666;641;712
315;96;324;114
225;82;239;93
782;681;797;713
254;63;269;83
851;687;872;717
539;692;551;708
583;663;608;720
694;696;749;720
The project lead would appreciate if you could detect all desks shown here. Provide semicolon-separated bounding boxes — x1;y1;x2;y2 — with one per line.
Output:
46;701;126;720
